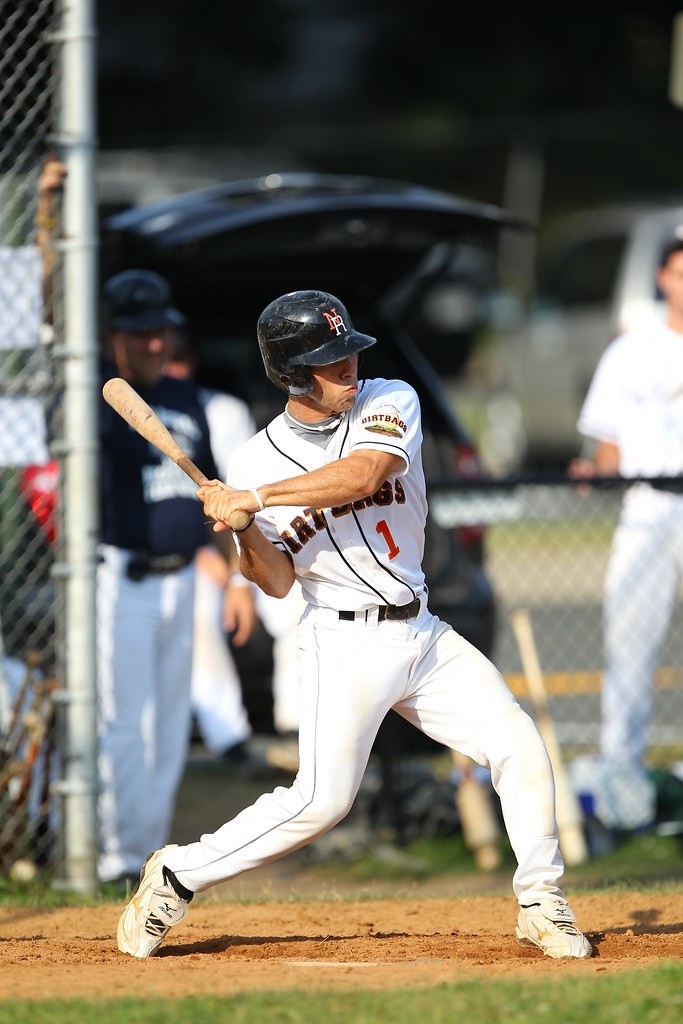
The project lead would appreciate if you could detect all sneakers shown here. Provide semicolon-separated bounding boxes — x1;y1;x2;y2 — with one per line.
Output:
117;842;190;957
515;898;593;960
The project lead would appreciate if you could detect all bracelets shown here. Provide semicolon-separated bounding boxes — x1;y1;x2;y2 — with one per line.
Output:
251;488;264;511
229;515;256;532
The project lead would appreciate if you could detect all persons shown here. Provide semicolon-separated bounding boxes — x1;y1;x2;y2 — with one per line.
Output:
94;271;258;886
163;313;290;784
564;221;682;859
116;290;595;962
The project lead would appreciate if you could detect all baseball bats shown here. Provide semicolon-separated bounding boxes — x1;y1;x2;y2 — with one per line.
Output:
447;750;499;872
103;377;247;532
511;610;591;870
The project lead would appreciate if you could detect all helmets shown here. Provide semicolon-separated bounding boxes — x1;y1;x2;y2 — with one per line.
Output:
99;269;186;331
258;289;377;399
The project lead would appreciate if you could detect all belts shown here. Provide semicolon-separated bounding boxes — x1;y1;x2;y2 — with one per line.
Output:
338;585;431;621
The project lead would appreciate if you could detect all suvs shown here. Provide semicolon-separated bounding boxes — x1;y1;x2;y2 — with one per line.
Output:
0;170;509;763
